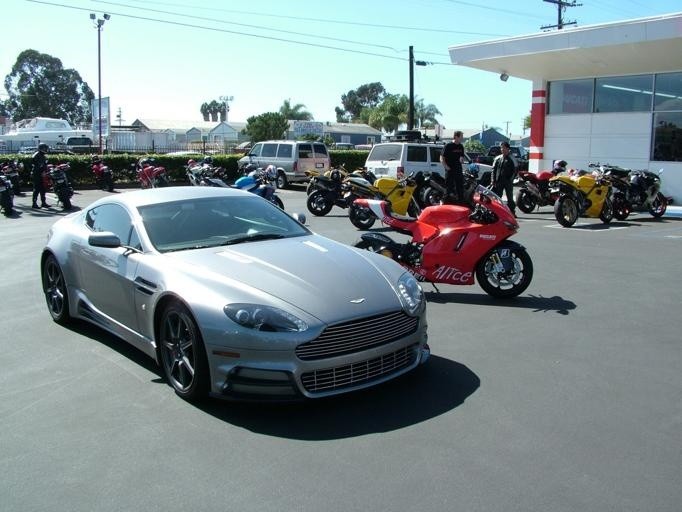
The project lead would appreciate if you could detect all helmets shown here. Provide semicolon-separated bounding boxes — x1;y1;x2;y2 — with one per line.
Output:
38;142;50;154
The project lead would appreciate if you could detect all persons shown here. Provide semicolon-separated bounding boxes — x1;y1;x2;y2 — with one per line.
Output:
31;143;51;209
439;131;465;202
491;142;518;219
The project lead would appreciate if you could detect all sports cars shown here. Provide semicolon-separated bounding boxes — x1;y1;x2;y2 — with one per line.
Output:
39;186;430;405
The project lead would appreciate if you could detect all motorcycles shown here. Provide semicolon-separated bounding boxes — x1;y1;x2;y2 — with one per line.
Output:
0;174;15;215
246;166;277;187
609;168;667;221
1;157;24;196
132;156;167;189
199;171;285;210
423;168;479;207
517;159;568;214
353;185;533;300
548;168;614;227
569;163;611;178
186;160;227;184
46;162;73;209
351;165;368;175
305;164;348;196
307;175;374;216
86;156;119;192
343;171;422;229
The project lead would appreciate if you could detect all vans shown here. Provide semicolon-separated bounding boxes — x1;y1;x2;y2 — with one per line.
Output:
238;138;328;189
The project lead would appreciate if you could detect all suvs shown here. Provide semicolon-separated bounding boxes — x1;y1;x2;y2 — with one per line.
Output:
366;131;495;187
64;137;101;154
